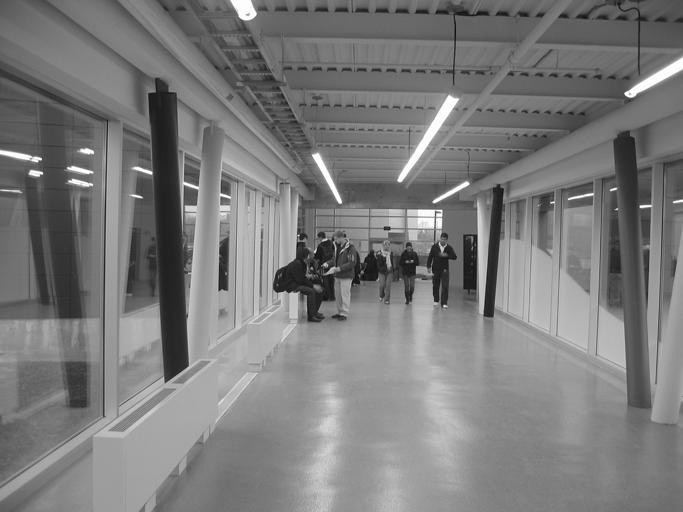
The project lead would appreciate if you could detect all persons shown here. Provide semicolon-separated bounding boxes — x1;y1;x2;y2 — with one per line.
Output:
376;240;396;304
364;250;377;281
306;248;320;281
127;228;137;296
322;229;357;321
182;231;189;268
145;236;157;297
426;232;457;308
610;240;621;274
297;233;308;248
219;236;229;291
399;242;419;305
284;248;325;323
310;231;336;302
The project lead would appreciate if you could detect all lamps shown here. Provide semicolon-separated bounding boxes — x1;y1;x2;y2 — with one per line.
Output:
396;2;462;186
431;149;473;205
618;0;683;99
307;94;343;207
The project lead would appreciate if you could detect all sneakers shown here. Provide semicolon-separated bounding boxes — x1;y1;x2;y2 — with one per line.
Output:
433;302;449;311
377;295;390;304
405;298;412;304
304;312;348;323
